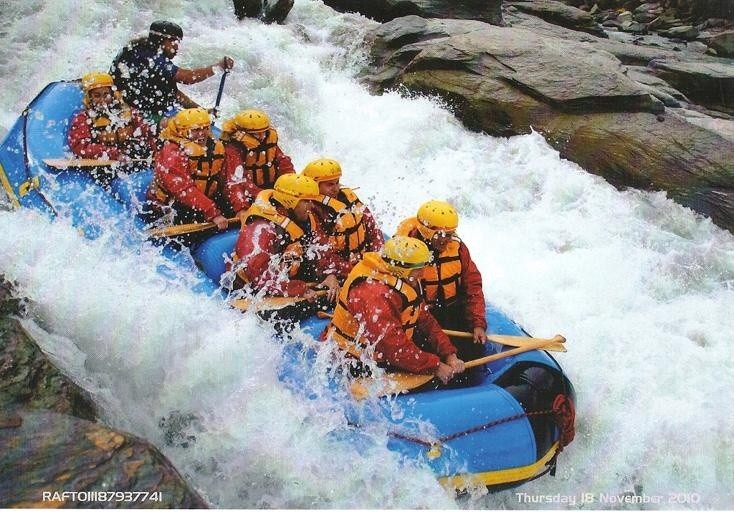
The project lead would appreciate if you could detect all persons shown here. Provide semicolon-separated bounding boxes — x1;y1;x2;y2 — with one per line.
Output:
216;110;295;210
301;159;384;279
66;73;157;184
108;21;234;120
395;201;488;368
225;172;341;329
324;235;464;392
140;106;237;242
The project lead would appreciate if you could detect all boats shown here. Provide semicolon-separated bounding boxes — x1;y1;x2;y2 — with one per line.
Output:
0;79;577;497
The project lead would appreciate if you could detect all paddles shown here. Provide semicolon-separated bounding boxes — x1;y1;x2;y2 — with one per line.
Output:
42;158;156;169
352;335;566;400
443;328;567;352
142;217;241;239
227;290;336;312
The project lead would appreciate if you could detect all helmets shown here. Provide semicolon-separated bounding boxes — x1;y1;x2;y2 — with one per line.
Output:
271;172;320;212
300;157;343;186
414;200;460;241
379;235;434;280
171;108;212;136
148;19;184;46
233;109;271;134
82;72;114;92
